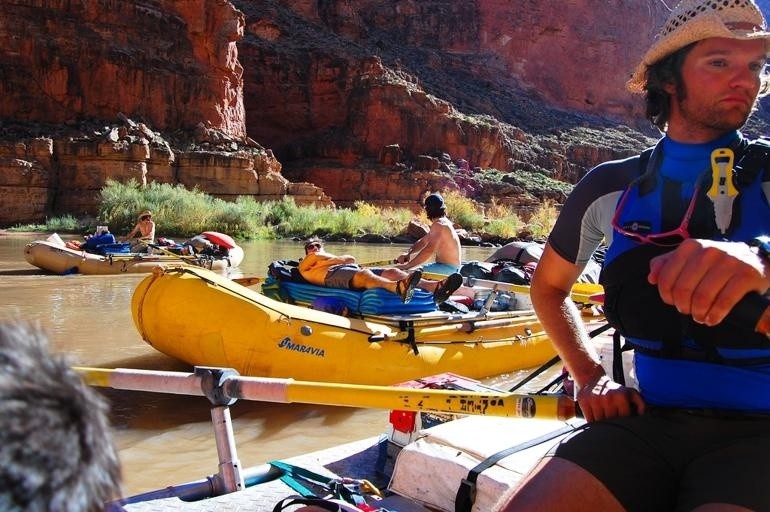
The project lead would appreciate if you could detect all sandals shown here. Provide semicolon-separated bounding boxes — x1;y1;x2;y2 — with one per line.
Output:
433;272;463;304
394;270;423;304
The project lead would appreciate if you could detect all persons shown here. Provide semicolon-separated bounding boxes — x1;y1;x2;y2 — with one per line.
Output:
0;304;125;511
396;193;463;277
66;230;116;254
494;0;770;512
120;209;156;253
298;237;463;307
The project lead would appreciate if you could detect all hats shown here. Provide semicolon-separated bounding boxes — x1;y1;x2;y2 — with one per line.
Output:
137;209;153;221
424;193;447;210
621;0;770;96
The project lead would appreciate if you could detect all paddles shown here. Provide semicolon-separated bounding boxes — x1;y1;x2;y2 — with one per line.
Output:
421;271;605;305
231;257;409;287
137;239;196;266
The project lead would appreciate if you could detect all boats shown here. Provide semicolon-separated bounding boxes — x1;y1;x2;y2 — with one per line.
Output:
23;230;245;275
130;261;560;385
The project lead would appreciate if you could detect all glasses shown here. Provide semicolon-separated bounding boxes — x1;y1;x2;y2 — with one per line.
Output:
305;243;322;250
609;177;702;248
